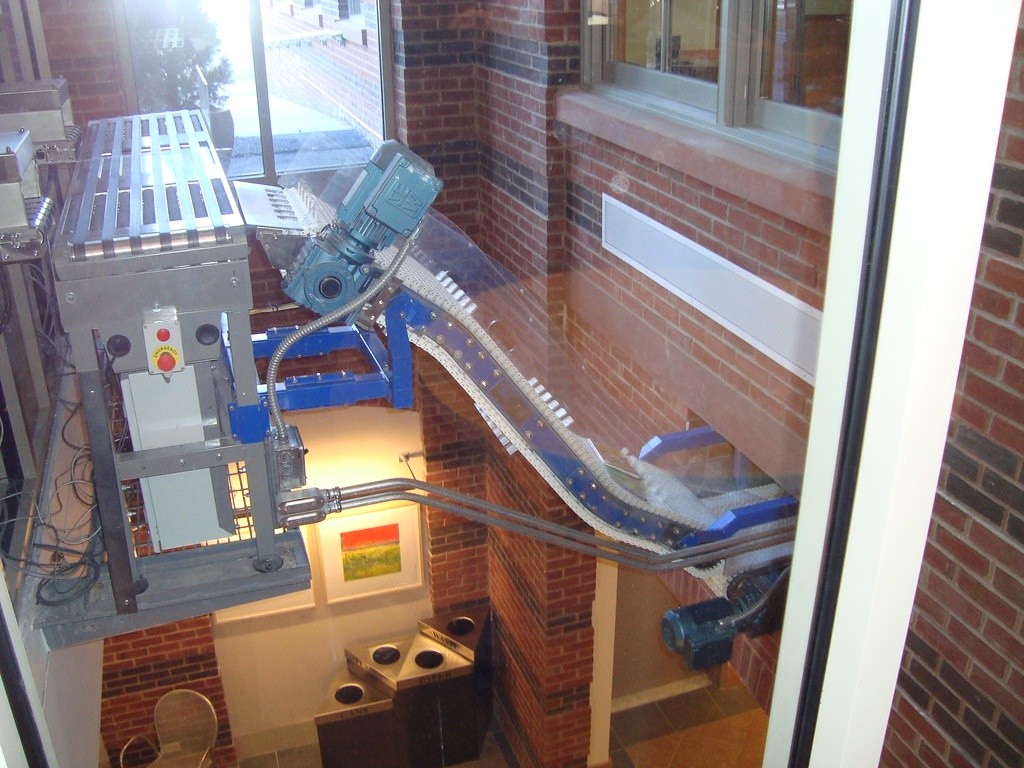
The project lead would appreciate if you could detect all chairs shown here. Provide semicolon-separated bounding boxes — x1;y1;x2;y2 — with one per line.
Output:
120;689;218;768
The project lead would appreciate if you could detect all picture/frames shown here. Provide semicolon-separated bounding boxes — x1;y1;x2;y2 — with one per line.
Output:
216;527;316;623
314;500;423;607
